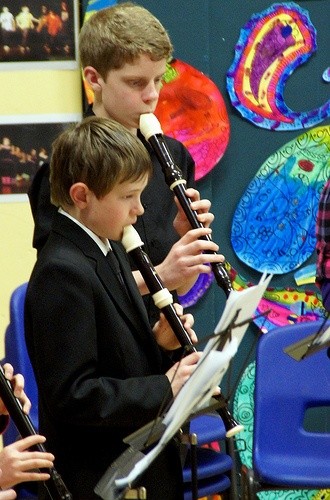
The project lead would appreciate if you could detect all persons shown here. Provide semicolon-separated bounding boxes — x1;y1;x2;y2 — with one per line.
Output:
2;3;80;191
27;2;226;360
0;362;55;500
24;116;226;500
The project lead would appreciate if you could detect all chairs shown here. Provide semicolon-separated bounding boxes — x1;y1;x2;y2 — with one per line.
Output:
5;283;330;500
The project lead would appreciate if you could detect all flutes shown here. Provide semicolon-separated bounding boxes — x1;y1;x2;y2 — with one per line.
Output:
139;112;235;299
121;226;245;439
0;365;70;500
